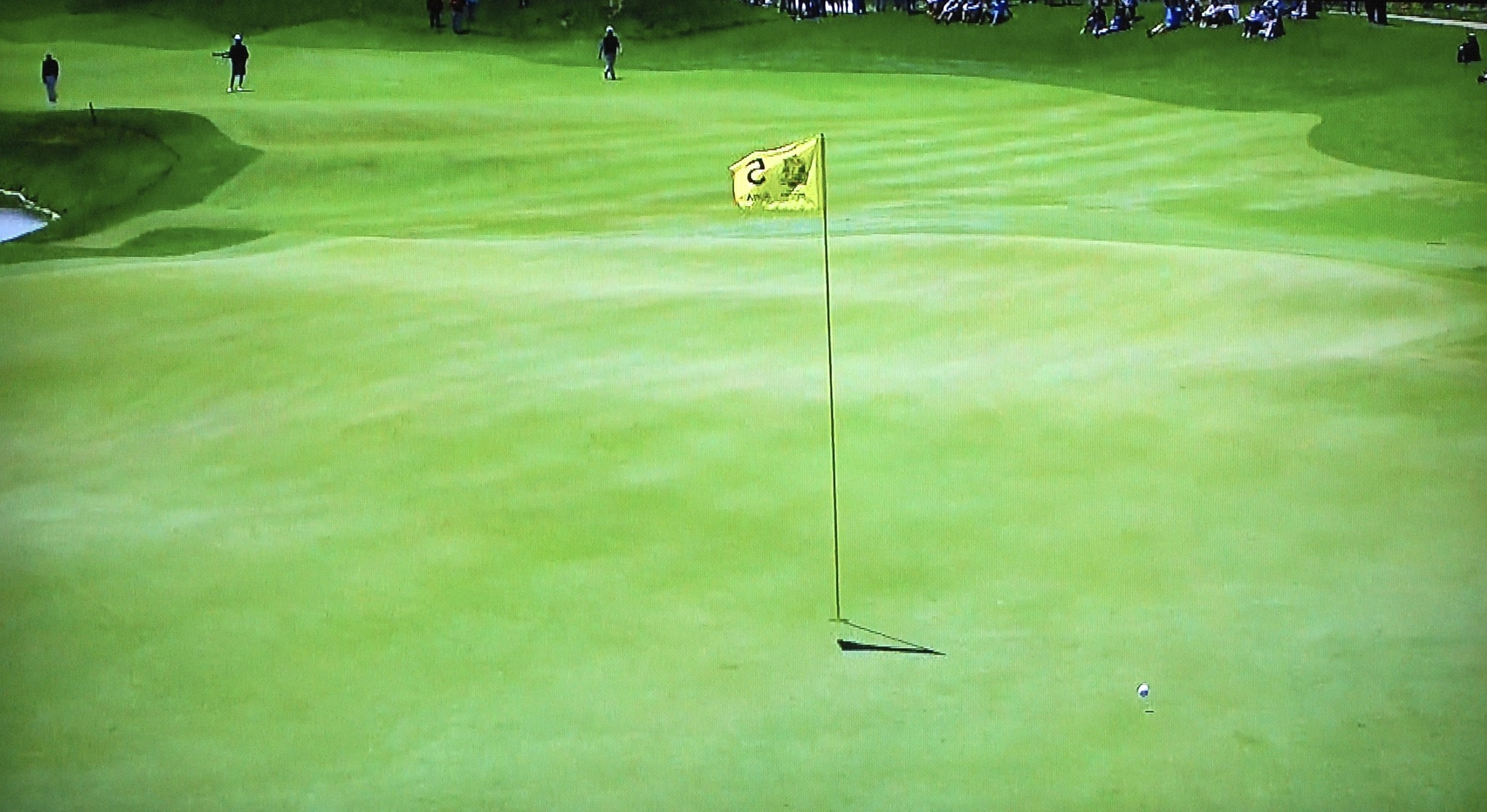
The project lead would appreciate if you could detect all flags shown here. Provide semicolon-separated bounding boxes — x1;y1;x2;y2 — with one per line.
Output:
729;134;825;211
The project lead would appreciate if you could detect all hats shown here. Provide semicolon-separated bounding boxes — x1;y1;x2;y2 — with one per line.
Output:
606;27;614;31
234;34;241;40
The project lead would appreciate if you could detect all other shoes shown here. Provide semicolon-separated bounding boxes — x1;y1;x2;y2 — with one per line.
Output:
605;71;608;79
228;87;232;93
237;86;243;91
612;75;615;80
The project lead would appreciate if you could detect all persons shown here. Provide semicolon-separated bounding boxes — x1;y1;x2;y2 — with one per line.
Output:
426;0;528;36
750;0;1487;85
223;34;250;92
40;52;60;102
598;26;623;80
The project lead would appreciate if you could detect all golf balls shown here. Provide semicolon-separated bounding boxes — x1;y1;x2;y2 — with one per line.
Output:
1136;683;1150;697
